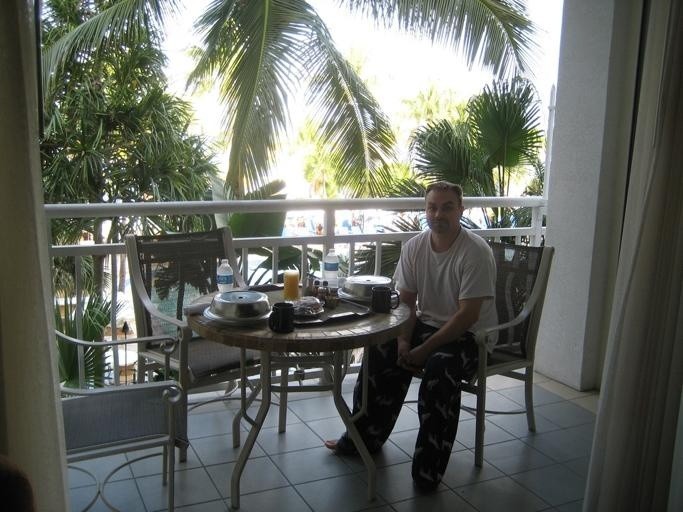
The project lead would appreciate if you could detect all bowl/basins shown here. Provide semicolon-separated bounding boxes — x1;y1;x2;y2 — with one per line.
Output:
204;290;271;323
338;275;400;301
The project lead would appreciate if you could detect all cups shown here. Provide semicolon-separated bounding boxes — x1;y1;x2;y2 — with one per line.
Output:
269;304;294;334
283;268;300;300
372;287;400;313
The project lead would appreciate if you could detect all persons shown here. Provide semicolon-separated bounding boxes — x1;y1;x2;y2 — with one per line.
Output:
325;181;499;493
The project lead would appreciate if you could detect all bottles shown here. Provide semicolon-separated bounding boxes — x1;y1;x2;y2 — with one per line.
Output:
216;258;234;292
313;248;341;310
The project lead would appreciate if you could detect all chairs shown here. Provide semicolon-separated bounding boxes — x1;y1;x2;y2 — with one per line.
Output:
123;228;292;461
402;239;554;470
55;324;185;509
